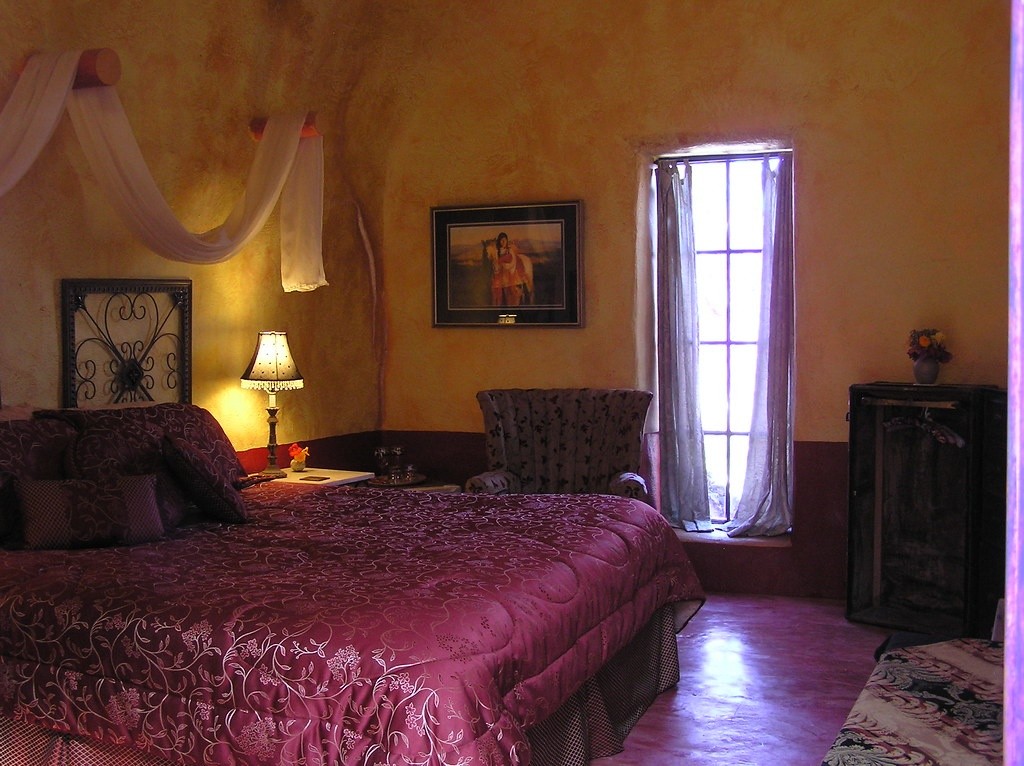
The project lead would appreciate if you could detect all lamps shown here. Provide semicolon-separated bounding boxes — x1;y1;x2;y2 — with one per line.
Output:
238;329;305;477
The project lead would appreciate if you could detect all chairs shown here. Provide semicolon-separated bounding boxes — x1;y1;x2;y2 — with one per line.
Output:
465;386;655;500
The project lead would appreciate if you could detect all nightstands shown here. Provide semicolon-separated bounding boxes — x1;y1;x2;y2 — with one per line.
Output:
245;466;374;490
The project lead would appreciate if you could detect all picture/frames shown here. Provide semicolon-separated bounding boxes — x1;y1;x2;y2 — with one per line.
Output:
431;198;586;328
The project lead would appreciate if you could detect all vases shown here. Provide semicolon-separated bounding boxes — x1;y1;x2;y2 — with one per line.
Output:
911;352;940;382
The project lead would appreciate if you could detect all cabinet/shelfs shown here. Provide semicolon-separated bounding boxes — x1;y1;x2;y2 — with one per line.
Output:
846;379;1006;641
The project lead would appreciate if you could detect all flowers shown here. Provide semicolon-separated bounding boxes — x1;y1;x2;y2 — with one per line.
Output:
906;327;952;363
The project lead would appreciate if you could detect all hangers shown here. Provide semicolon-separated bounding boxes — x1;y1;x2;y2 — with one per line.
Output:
882;403;965;448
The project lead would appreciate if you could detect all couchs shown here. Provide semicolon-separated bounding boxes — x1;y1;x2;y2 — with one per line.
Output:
822;632;1003;766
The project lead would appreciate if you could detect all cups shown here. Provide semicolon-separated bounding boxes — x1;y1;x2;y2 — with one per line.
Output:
403;464;417;483
390;464;405;485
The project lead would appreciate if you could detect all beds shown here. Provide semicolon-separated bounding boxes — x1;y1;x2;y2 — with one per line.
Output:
1;400;707;766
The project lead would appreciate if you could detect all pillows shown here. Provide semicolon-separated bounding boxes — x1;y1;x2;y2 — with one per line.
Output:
1;419;75;539
34;402;249;493
12;475;166;546
162;433;247;524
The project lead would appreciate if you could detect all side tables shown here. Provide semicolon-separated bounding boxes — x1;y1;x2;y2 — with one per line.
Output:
360;472;460;492
